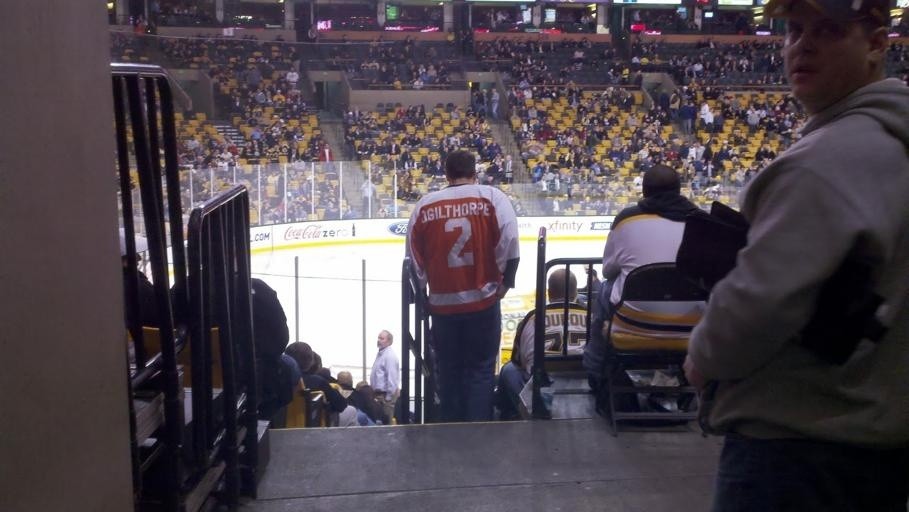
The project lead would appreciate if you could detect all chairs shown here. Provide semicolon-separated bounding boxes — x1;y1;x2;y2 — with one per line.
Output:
588;261;714;437
139;320;343;428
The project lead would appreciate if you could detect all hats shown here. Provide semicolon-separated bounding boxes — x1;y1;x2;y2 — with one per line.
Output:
760;0;897;30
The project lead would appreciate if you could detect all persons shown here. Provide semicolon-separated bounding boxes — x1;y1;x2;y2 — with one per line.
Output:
682;1;908;511
580;267;601;291
109;3;909;234
120;233;401;427
583;164;704;401
496;268;593;420
408;150;521;422
